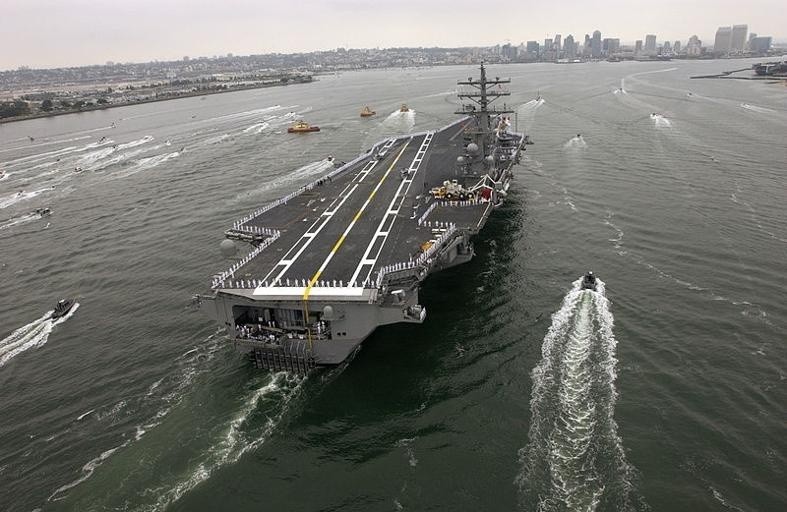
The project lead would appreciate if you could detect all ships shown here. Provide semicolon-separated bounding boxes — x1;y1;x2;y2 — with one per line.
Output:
190;62;534;376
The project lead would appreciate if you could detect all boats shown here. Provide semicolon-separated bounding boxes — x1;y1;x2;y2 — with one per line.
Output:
359;106;376;117
580;271;598;293
400;104;409;112
51;296;75;318
287;121;320;133
